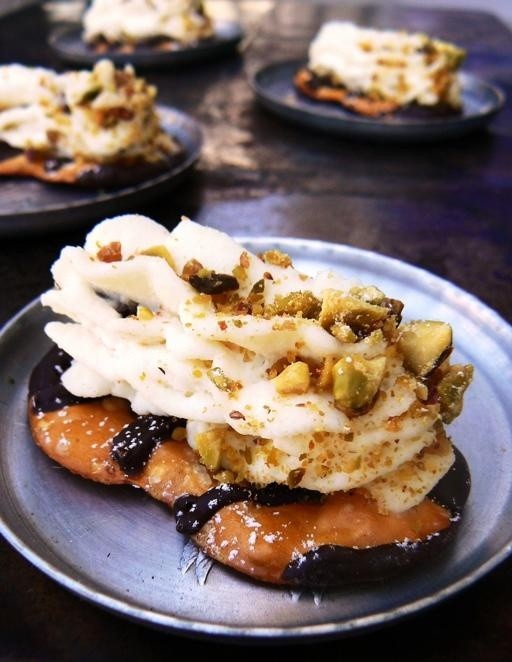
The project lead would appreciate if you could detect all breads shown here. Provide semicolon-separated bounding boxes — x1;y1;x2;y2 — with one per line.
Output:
28;346;472;590
294;66;461;121
84;37;186;60
0;131;184;187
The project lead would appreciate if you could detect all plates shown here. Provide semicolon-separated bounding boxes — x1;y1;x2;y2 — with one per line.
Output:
45;7;246;71
0;96;207;237
244;42;507;144
0;233;512;640
